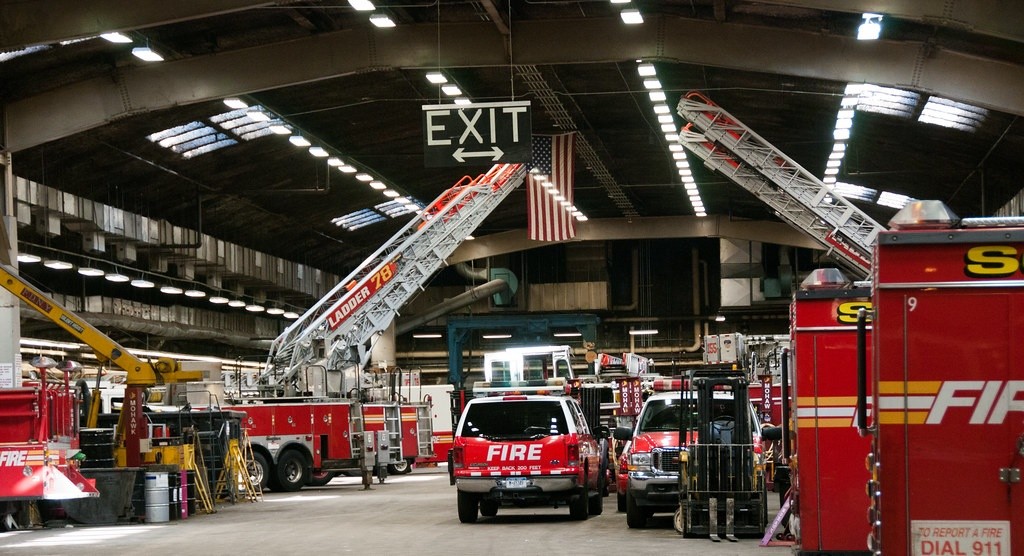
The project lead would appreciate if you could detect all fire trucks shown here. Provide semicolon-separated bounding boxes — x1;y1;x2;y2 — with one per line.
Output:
0;163;524;499
675;88;1024;555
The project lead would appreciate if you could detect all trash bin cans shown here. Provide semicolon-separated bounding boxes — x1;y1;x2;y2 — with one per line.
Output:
145;472;169;523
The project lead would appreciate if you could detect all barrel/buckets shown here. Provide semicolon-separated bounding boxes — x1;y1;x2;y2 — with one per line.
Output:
132;467;196;522
79;428;115;469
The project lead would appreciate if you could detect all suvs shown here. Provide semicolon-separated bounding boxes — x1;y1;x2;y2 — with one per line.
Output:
451;379;610;523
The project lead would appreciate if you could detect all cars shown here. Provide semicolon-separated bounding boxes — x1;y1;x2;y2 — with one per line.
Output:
613;377;781;529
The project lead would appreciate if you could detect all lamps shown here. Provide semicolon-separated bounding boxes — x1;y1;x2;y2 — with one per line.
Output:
369;13;396;28
637;65;708;217
19;252;298;322
100;31;134;44
530;169;588;222
426;71;448;84
824;86;866;184
857;22;882;40
441;83;462;96
347;0;376;11
132;46;164;62
224;97;423;214
621;9;644;25
454;97;471;103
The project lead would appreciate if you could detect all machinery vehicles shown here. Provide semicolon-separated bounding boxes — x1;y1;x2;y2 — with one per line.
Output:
0;260;211;522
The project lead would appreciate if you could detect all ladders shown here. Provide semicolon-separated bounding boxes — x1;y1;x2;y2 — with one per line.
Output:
238;427;265;501
214;440;259;504
194;463;214;514
